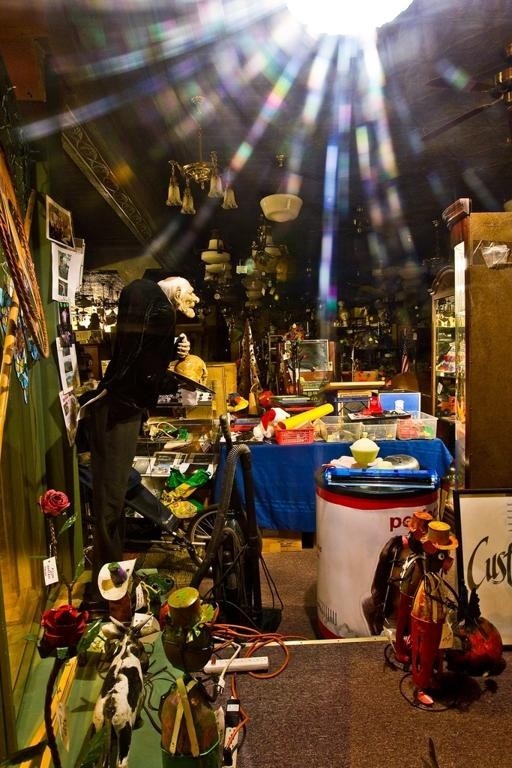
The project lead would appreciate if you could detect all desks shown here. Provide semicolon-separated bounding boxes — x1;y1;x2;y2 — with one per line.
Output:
220;438;441;639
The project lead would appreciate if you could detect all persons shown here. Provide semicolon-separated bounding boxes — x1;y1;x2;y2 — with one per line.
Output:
361;512;507;712
88;275;202;593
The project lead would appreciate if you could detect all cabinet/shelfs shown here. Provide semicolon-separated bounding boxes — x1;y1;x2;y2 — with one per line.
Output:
429;264;456;425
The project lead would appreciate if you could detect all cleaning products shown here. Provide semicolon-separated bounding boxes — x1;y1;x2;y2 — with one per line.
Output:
260;407;292;431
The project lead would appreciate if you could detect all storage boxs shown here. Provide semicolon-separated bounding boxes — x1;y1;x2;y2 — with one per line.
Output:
318;410;439;443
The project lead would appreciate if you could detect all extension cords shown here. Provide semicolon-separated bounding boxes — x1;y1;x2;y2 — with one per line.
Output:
203;657;268;674
222;699;240;768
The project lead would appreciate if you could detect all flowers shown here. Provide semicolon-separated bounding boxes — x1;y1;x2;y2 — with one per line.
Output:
2;489;104;767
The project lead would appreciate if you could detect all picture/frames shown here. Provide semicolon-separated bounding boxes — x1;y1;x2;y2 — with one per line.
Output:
453;490;512;651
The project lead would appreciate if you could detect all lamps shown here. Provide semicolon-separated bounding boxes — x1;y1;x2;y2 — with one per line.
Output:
165;95;305;308
